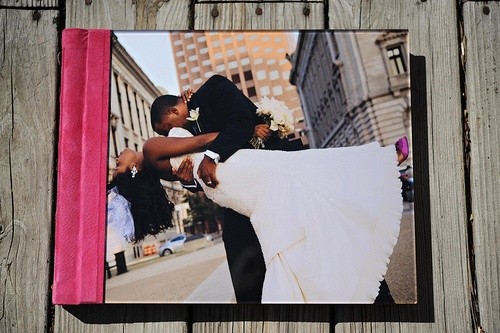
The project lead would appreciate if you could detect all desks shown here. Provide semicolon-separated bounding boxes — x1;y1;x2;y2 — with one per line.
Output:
0;0;500;333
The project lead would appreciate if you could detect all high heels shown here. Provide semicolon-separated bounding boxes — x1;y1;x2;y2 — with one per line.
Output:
395;136;409;166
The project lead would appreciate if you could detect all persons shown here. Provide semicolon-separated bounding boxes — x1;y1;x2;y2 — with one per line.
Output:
149;73;400;304
108;123;411;303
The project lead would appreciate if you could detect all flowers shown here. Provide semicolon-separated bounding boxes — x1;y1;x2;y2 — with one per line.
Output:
248;96;295;149
186;106;202;132
